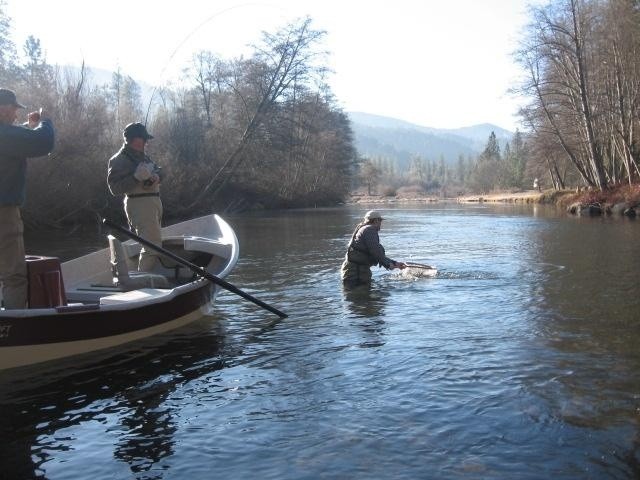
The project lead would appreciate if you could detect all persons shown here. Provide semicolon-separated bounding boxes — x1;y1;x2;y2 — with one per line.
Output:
533;177;538;194
539;178;545;193
1;88;57;310
555;175;560;188
106;122;163;275
342;209;407;286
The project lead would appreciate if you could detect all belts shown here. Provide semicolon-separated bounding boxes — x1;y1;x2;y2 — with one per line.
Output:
127;192;159;198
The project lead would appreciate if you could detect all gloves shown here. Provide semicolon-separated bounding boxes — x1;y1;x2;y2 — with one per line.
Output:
134;161;154;182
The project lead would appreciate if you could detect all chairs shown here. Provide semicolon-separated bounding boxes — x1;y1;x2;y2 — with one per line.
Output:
106;234;169;290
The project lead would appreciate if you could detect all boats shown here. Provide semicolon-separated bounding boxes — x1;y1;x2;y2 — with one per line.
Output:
1;215;239;383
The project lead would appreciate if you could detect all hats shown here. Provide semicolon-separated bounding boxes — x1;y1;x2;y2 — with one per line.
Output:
364;210;387;220
0;88;28;109
123;122;154;140
